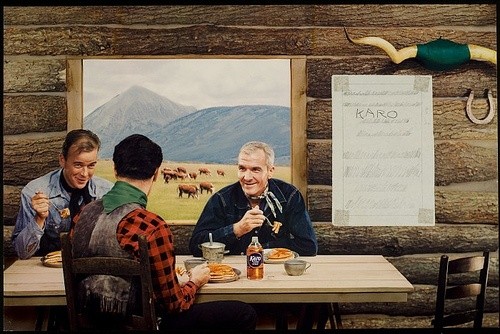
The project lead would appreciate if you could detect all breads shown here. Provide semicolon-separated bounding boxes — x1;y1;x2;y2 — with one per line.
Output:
272;221;283;234
60;208;70;219
44;249;65;264
266;248;293;259
208;264;237;281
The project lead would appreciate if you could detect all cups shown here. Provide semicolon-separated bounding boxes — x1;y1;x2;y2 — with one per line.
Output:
198;233;230;263
185;258;206;271
284;260;311;276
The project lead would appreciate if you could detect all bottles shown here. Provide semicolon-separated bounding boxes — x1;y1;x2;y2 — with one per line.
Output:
246;237;263;279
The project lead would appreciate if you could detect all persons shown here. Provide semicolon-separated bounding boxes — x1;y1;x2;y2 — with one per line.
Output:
67;134;212;316
10;129;115;260
189;141;318;257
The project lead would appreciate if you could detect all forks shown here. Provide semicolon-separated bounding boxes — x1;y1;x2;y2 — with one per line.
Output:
265;216;275;229
36;191;69;219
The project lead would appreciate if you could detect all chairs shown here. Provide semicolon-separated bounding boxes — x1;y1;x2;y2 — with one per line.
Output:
59;233;157;333
434;252;491;333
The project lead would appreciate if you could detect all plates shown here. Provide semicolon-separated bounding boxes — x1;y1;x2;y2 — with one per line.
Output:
263;248;299;264
209;268;242;283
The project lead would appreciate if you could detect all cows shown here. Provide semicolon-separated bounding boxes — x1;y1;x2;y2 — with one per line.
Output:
217;169;225;176
177;184;196;198
200;182;215;194
189;184;200;198
198;167;211;176
161;166;197;184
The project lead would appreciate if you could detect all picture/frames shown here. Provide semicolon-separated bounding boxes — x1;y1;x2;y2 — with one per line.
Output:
65;55;308;226
